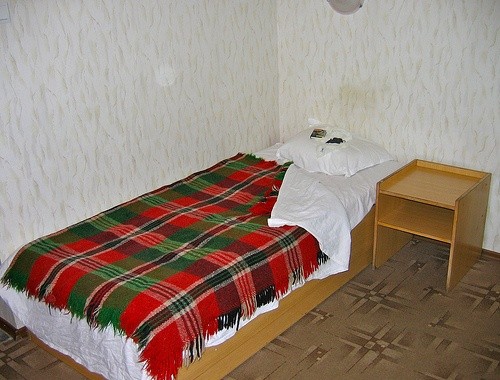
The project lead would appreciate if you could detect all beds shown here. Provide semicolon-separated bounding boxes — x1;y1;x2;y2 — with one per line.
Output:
0;141;402;380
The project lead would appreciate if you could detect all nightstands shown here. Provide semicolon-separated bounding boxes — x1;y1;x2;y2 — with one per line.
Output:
372;158;492;295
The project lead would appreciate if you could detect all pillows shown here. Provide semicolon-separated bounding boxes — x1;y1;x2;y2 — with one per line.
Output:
275;117;396;178
